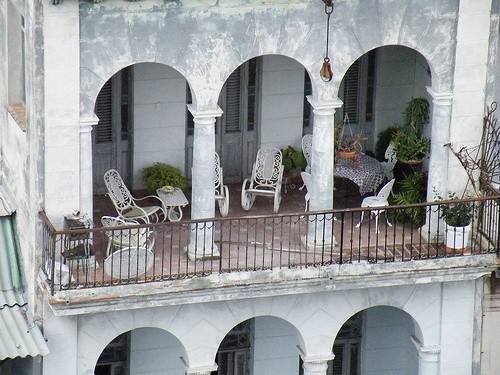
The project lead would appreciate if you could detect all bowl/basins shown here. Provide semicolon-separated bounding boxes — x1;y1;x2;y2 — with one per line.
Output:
161;186;174;192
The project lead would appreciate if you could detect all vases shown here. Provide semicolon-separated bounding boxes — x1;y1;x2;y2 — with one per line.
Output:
336;150;356;158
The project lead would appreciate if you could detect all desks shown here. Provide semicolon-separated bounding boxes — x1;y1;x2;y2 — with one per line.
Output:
334;155;384;208
156;187;189;221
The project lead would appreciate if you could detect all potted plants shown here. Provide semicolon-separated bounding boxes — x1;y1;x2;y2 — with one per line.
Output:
142;161;189;206
276;145;305;186
391;98;431;179
429;187;474;250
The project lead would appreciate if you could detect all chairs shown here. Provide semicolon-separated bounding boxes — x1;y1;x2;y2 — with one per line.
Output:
241;146;285;213
298;134;312;213
379;144;397;198
101;215;156;256
215;151;229;217
104;169;167;224
355;178;395;234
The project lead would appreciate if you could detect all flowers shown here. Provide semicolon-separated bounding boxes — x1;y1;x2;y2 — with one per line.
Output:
335;123;368;161
